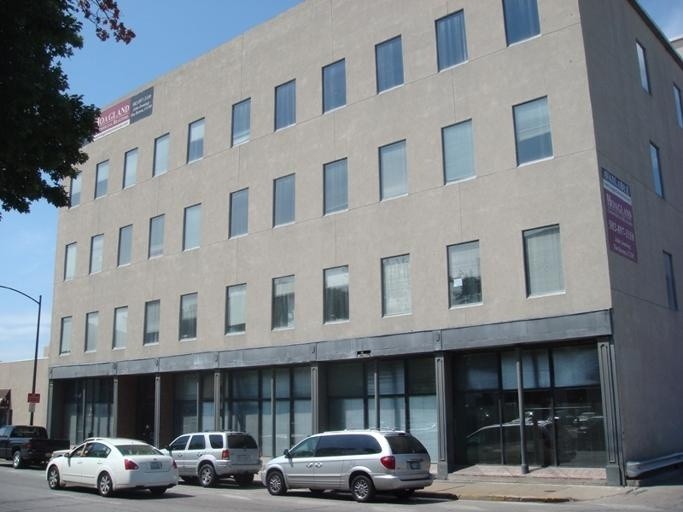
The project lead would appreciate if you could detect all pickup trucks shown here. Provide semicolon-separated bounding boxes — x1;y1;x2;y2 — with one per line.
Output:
0;424;70;470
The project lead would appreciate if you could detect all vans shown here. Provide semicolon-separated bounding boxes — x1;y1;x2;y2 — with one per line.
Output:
463;419;578;467
260;427;435;505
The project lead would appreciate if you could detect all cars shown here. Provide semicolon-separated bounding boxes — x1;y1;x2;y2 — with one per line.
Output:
49;437;112;461
511;408;605;452
45;437;180;498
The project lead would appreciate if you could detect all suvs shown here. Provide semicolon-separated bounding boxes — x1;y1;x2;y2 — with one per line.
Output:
158;431;263;489
